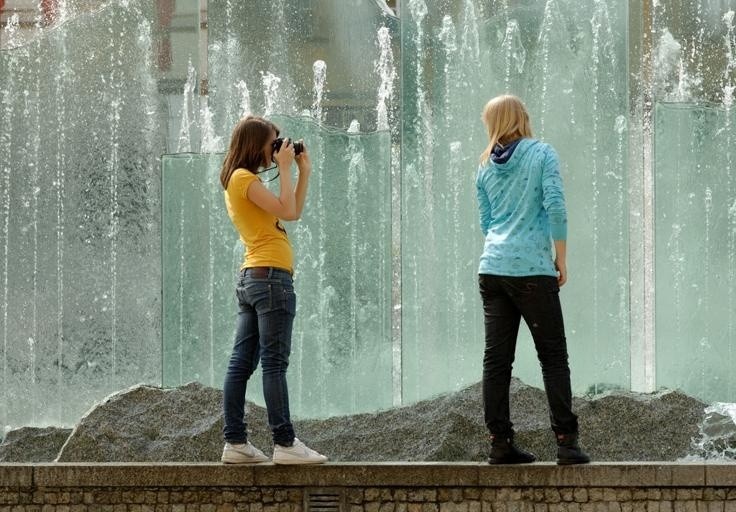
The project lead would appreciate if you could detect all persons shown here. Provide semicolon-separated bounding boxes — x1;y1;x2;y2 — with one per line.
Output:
216;114;327;468
474;92;591;468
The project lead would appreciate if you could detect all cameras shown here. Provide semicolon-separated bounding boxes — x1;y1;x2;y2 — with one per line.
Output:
271;137;305;163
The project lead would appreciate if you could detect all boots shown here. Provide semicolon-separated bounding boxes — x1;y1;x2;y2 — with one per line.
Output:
488;432;537;465
552;432;591;465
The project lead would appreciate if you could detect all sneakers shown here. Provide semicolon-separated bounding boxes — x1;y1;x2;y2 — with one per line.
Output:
219;441;270;464
272;440;328;469
